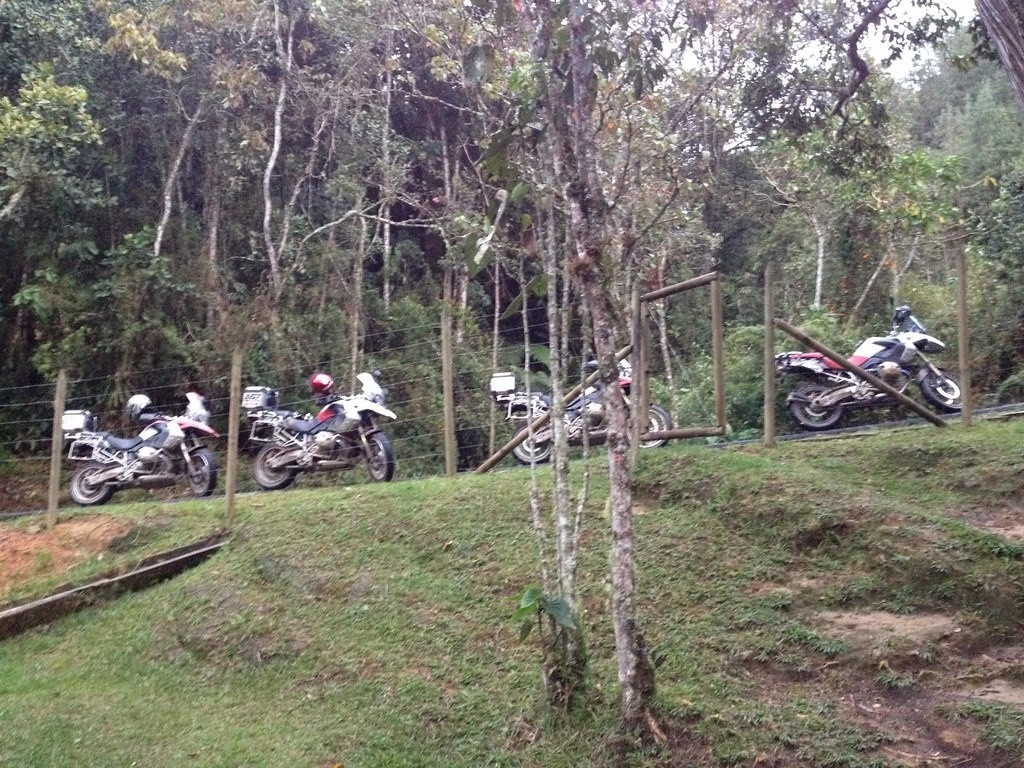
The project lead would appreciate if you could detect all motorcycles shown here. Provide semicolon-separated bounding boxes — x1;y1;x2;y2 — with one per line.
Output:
60;397;221;505
240;370;398;491
488;352;673;467
775;305;962;431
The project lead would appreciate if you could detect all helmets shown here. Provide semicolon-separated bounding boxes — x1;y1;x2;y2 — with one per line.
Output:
310;373;335;396
892;306;911;325
125;394;152;423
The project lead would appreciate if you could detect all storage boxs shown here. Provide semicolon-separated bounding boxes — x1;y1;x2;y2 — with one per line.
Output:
241;385;276;411
60;410;94;435
489;372;515;398
